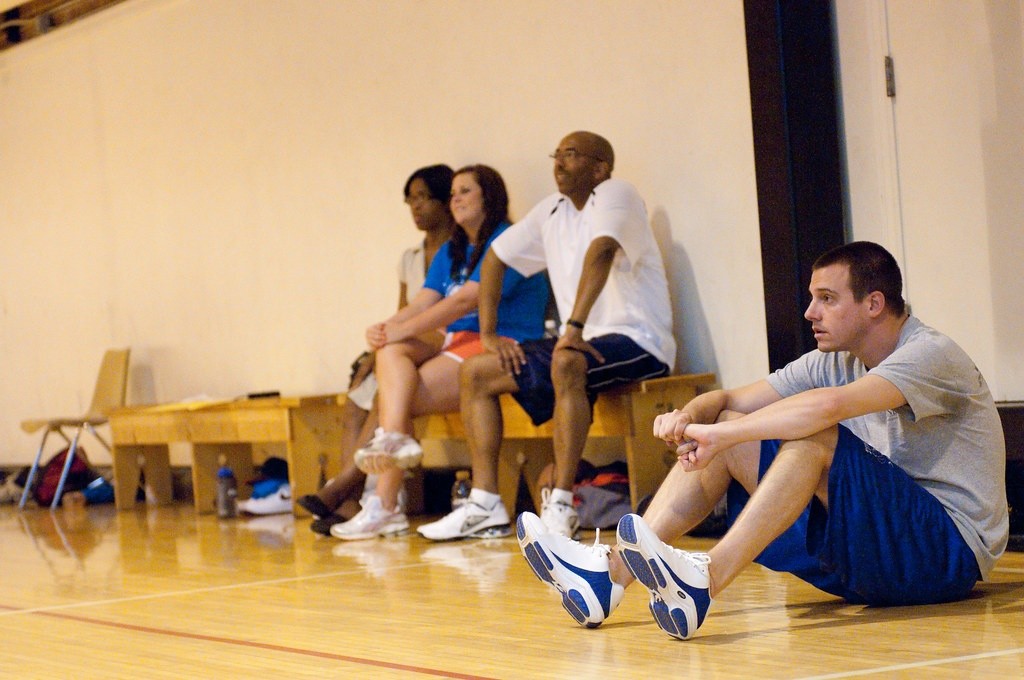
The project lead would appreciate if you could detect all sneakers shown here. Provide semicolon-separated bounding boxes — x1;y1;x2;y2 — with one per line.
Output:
353;426;424;474
516;511;625;628
330;496;410;540
540;487;581;540
616;513;713;640
417;496;512;541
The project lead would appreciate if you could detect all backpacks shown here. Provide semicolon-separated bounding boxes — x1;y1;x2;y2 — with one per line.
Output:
34;449;95;506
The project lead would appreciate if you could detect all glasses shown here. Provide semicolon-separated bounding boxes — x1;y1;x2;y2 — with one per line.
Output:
546;149;604;165
403;192;435;205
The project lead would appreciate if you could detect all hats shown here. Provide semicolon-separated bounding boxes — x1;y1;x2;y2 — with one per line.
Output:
243;457;290;485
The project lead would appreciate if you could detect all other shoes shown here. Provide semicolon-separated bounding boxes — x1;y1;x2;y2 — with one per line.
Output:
310;494;362;533
296;494;343;518
236;482;293;515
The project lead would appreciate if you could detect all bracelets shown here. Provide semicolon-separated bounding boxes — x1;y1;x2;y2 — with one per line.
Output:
567;319;584;329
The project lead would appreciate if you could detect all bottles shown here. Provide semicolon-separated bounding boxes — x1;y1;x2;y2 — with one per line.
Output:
451;470;473;511
215;468;238;519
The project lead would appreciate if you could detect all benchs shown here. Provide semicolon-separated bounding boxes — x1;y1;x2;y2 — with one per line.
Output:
110;395;349;519
405;372;717;522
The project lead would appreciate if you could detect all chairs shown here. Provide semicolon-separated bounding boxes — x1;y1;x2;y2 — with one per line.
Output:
19;348;130;512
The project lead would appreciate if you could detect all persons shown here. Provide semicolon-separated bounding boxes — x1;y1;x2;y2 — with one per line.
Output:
297;164;548;540
416;132;677;541
516;241;1009;641
